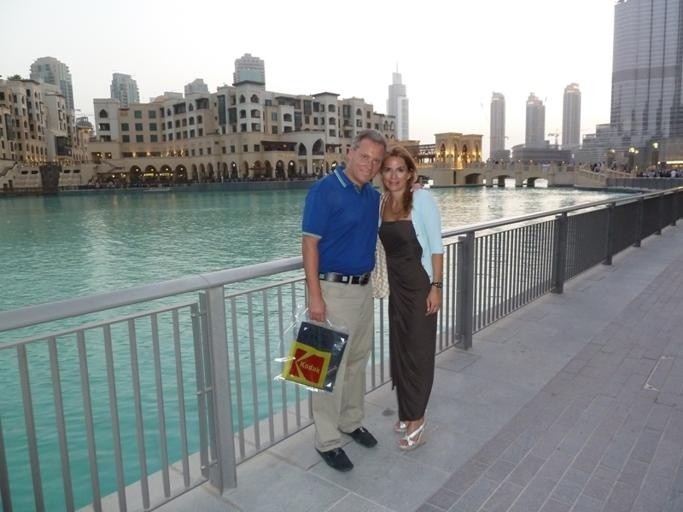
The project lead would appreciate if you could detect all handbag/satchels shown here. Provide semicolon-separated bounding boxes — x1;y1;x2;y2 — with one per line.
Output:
372;238;391;299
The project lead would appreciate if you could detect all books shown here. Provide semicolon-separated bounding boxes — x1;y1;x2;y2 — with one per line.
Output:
287;321;349;394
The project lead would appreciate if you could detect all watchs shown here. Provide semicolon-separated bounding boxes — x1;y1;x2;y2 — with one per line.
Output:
433;281;442;288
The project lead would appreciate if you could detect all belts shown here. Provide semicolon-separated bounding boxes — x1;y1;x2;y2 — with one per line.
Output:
316;273;370;286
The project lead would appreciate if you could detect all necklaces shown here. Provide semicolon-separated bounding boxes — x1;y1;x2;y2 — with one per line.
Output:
388;197;404;215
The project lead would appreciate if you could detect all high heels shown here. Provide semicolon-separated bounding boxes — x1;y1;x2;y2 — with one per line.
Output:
394;410;429;450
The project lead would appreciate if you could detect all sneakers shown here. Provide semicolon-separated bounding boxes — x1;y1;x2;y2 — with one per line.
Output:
337;425;379;448
314;443;355;471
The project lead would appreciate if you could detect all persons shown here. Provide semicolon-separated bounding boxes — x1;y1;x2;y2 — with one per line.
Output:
373;145;445;450
637;167;682;178
300;130;423;472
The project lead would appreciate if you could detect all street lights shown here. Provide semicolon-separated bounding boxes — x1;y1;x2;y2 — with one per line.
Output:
605;142;662;177
